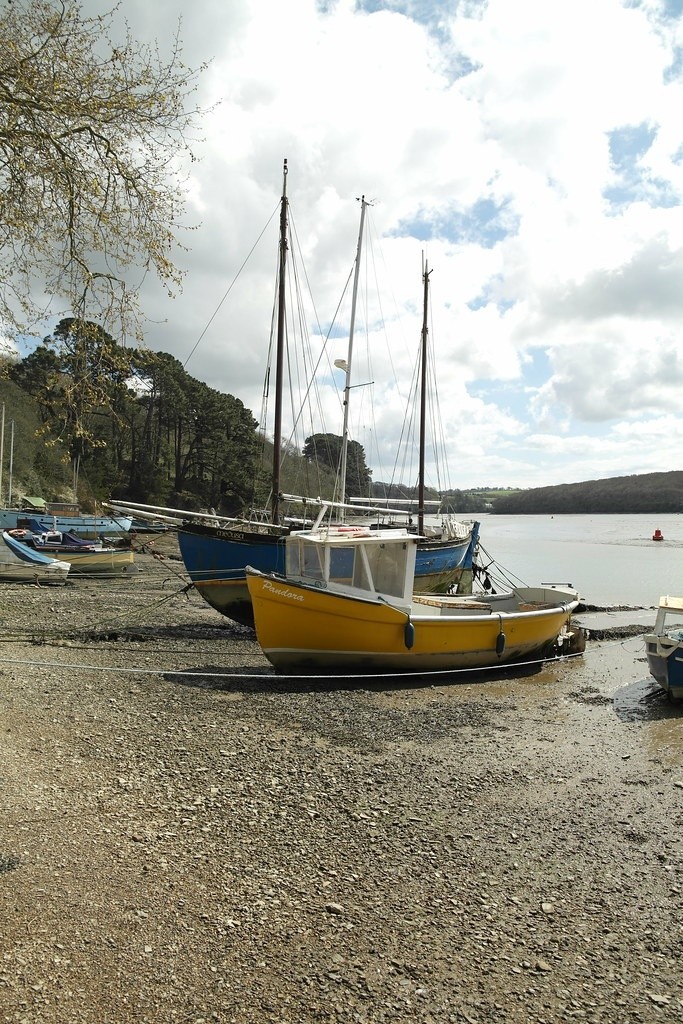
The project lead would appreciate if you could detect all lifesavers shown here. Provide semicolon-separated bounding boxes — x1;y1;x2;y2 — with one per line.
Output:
8;528;27;538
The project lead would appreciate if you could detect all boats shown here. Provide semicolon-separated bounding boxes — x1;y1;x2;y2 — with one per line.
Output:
0;400;173;586
642;594;683;705
242;524;584;675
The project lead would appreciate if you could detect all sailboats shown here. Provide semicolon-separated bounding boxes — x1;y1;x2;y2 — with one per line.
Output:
95;155;481;630
